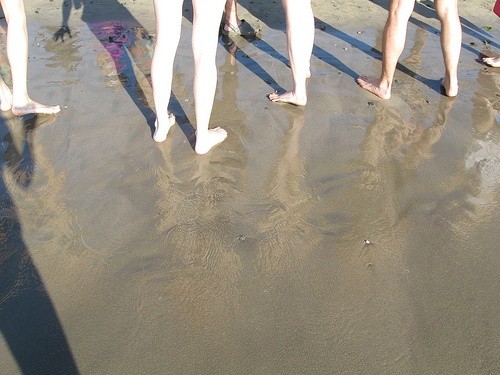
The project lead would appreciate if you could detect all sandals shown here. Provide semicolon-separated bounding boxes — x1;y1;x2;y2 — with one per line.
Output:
222;18;256;35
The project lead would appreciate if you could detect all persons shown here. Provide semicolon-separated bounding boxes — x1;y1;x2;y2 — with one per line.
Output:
0;0;61;115
269;0;315;105
222;0;263;35
356;0;463;99
482;0;500;68
150;0;229;154
